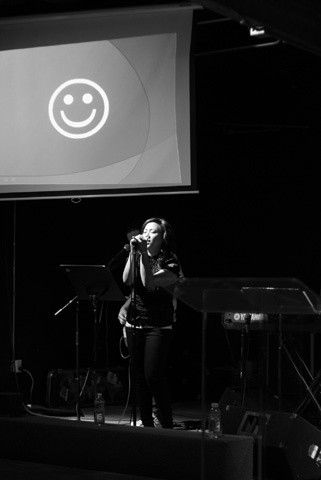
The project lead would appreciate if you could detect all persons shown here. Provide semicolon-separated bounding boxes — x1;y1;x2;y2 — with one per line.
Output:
117;217;185;430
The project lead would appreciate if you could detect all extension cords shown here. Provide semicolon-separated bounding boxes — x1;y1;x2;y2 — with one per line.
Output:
12;359;23;372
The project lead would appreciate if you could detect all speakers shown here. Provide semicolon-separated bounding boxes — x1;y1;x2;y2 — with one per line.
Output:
238;409;321;480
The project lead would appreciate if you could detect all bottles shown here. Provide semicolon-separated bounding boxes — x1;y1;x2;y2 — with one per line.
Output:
93;392;105;424
209;403;220;439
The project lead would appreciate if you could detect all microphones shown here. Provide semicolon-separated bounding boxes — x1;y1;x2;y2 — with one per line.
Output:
110;244;130;263
132;237;148;247
